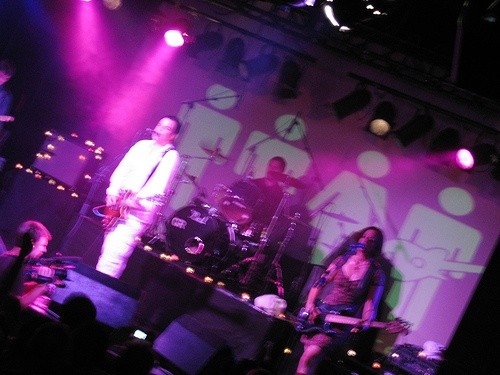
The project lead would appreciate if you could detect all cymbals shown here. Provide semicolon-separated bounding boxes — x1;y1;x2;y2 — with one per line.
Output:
271;170;308;190
200;145;227;160
276;214;313;228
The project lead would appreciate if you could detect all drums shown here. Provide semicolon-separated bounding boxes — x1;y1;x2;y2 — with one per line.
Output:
222;180;262;225
165;205;234;270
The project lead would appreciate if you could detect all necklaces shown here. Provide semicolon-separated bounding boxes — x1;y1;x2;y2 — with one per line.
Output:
353;254;366;270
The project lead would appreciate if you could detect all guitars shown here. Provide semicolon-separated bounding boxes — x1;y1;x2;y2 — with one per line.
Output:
296;298;408;336
102;191;167;229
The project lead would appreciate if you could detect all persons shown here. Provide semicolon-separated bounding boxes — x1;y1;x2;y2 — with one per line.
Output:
95;114;181;281
229;156;287;227
0;217;155;375
295;226;388;375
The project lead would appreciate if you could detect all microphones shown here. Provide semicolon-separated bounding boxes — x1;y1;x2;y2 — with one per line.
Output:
349;243;366;248
146;128;161;136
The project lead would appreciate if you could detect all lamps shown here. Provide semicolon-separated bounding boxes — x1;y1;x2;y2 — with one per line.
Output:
332;81;500;184
164;10;302;101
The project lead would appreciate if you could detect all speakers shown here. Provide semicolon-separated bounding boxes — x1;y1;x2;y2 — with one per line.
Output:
48;259;137;340
152;315;235;375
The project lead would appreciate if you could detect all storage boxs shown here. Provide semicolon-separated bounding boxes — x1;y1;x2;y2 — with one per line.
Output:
30;133;95;189
